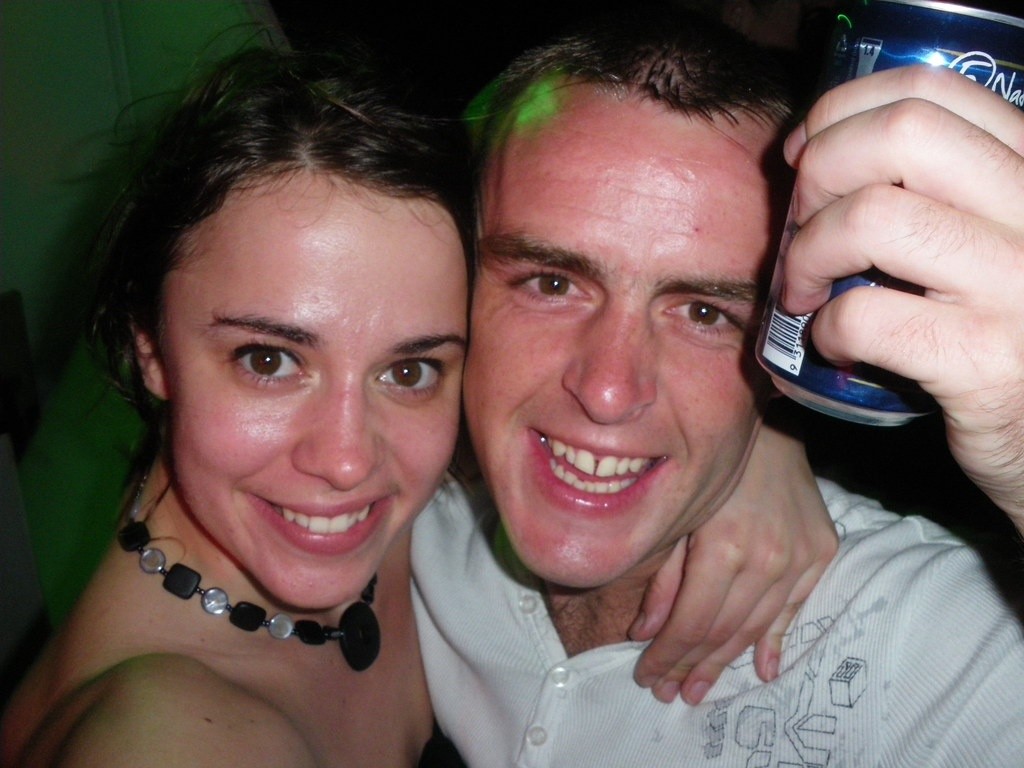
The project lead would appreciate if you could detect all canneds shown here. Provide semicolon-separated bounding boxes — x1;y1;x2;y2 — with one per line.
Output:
754;0;1024;428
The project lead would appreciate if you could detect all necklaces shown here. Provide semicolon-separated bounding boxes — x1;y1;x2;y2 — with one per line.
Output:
116;469;382;673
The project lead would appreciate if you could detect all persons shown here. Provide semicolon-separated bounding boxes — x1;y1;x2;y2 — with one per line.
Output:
0;50;841;768
408;24;1024;768
